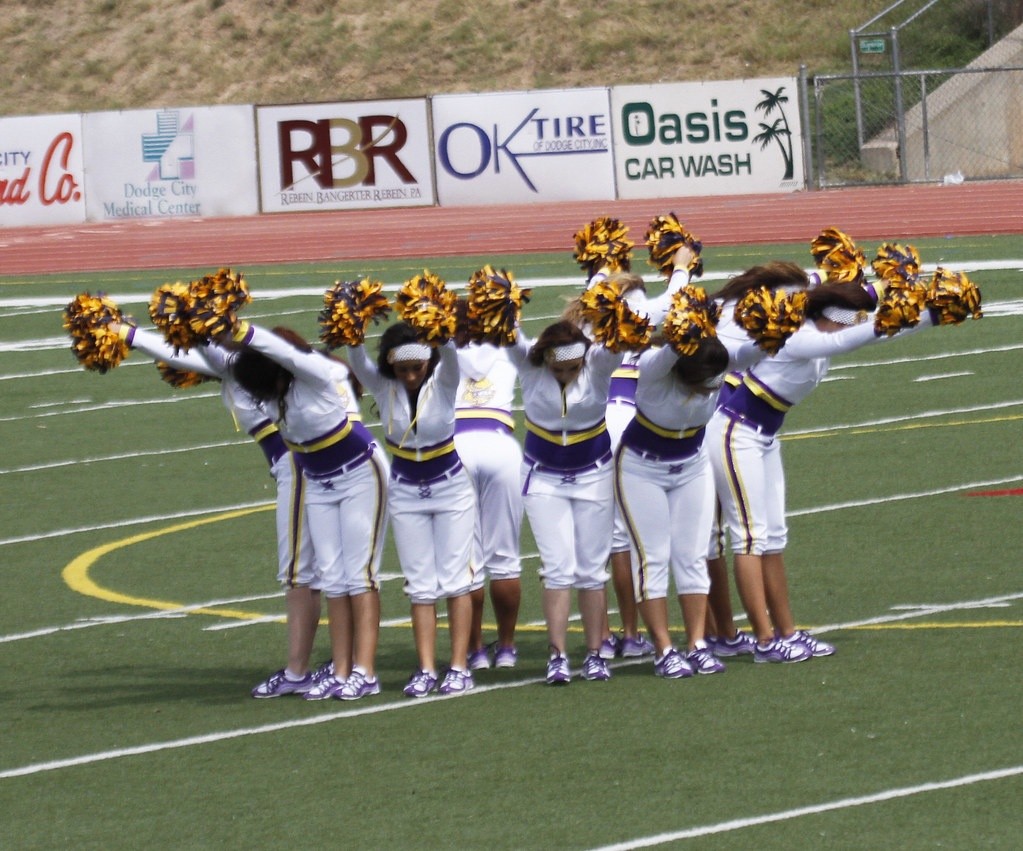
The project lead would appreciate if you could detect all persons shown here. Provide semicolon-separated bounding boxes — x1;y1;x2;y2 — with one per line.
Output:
705;224;985;664
569;206;808;679
62;258;659;704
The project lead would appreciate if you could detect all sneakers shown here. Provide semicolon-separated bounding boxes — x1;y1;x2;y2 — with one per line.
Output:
403;666;439;698
466;643;490;670
580;648;609;680
332;664;380;701
705;629;759;658
546;644;570;684
488;641;518;668
774;628;836;657
598;631;621;659
438;666;474;695
754;636;811;664
653;645;693;679
310;657;336;685
303;675;348;700
251;667;315;698
619;627;655;658
682;640;724;674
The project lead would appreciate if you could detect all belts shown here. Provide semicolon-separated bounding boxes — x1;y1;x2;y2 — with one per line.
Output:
305;444;376;480
720;407;774;436
390;462;463;486
626;443;699;462
522;451;613;497
